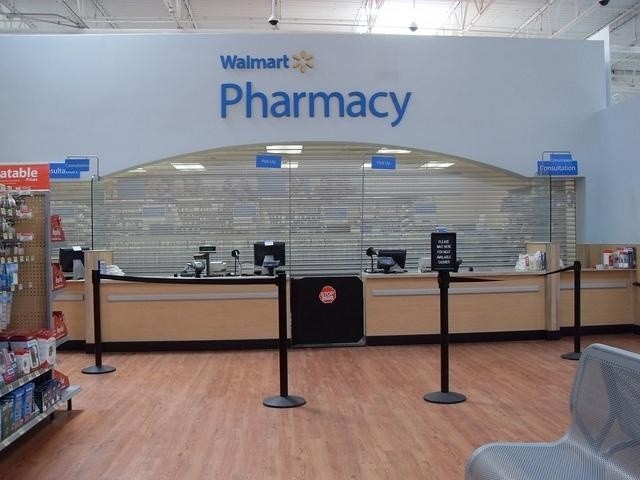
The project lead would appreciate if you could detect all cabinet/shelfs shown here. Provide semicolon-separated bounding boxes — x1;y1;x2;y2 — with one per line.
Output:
0;180;85;458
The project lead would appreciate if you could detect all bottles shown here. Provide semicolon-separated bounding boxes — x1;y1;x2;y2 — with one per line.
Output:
603;246;636;268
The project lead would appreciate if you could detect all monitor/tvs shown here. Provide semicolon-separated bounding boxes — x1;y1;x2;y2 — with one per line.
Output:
254;241;285;276
377;249;406;269
59;247;89;280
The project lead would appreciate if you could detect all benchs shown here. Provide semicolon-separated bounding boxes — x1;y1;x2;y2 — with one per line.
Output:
462;341;640;480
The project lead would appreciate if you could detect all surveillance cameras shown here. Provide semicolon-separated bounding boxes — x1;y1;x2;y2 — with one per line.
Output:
269;16;278;25
409;24;417;31
599;0;609;6
